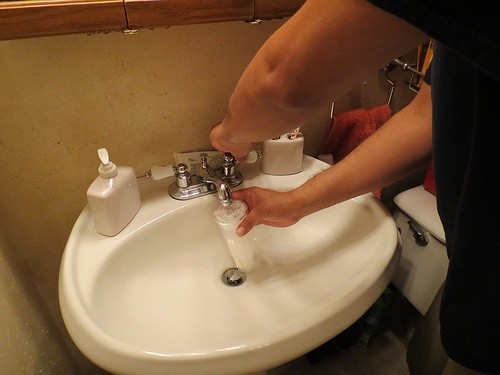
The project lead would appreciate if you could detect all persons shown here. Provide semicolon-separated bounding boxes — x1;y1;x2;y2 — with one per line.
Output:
209;0;500;375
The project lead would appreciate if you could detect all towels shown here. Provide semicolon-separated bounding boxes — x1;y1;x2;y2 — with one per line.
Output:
319;103;391;202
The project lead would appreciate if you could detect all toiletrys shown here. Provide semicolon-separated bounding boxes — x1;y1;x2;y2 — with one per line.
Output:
85;147;142;237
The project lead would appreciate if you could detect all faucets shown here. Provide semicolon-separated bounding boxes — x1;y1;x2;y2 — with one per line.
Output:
167;152;243;200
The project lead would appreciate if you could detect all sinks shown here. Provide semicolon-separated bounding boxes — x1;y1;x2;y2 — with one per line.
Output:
56;149;403;375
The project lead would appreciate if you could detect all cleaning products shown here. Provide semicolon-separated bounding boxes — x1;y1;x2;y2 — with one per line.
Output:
210;197;264;275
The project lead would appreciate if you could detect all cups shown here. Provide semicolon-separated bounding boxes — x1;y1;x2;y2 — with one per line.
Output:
213;198;261;274
